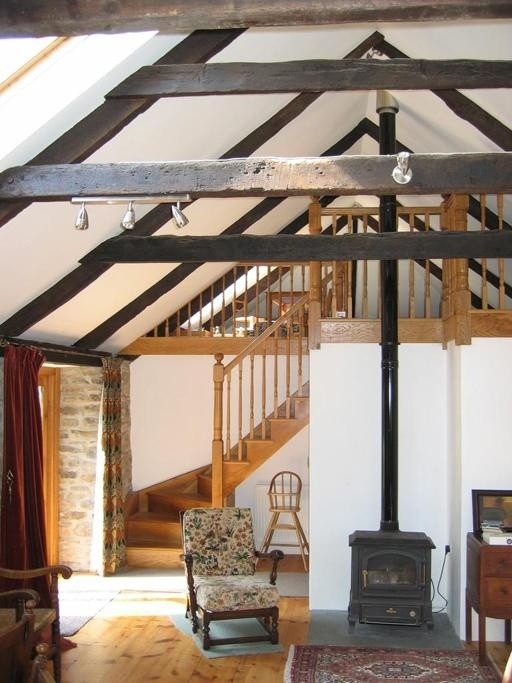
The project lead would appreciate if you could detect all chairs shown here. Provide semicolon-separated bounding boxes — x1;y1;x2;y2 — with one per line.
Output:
1;617;34;683
259;471;308;572
180;506;285;650
0;564;75;682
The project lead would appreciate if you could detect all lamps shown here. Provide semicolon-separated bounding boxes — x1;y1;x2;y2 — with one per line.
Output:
390;150;414;186
72;194;191;230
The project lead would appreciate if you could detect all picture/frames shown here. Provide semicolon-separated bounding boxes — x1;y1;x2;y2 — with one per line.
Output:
473;490;512;532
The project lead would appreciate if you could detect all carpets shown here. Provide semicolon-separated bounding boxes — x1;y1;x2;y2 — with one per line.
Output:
57;566;309;659
284;643;503;683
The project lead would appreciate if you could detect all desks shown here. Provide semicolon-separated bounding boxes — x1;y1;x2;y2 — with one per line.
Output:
266;290;310;328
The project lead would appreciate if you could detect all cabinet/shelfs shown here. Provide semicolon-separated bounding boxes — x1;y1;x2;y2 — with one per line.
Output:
466;532;512;666
